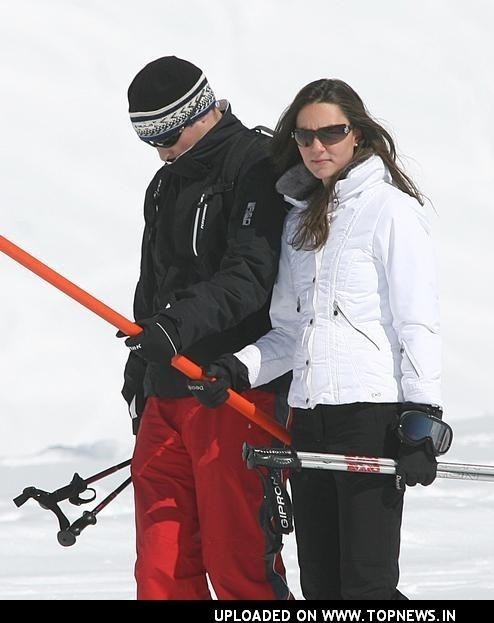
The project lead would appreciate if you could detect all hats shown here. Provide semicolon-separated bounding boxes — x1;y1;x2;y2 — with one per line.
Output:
128;56;216;141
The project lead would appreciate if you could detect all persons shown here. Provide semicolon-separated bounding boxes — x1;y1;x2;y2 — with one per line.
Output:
186;80;443;601
115;55;300;602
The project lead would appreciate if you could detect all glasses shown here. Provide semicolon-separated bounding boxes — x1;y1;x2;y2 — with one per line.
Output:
398;411;453;454
145;89;205;148
290;124;353;147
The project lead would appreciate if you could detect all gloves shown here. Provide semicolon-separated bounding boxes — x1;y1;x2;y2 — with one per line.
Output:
397;442;437;496
187;364;232;408
116;315;181;358
